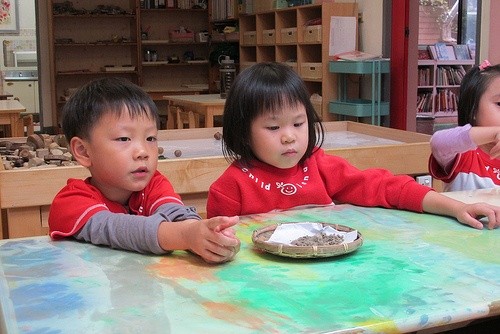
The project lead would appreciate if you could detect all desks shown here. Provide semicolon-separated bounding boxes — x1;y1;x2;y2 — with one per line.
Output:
0;99;26;137
163;93;226;128
1;120;431;239
0;187;500;333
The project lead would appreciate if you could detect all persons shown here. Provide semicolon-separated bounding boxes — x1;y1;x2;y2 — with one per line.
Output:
206;61;500;230
428;59;500;192
48;76;241;264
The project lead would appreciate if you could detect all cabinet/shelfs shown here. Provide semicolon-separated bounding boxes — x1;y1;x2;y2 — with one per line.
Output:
416;44;475;135
35;0;390;135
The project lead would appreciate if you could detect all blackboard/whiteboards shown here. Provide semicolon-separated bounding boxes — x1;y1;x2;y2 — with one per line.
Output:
328;16;356;56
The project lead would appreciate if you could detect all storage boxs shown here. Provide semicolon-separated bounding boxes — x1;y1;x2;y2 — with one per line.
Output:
243;31;257;44
300;63;323;80
280;27;297;42
262;29;276;43
303;26;321;42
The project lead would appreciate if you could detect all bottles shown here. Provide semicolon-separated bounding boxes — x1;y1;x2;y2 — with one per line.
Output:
218;55;236;99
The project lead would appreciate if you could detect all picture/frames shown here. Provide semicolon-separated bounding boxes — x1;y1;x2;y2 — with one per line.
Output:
0;0;20;36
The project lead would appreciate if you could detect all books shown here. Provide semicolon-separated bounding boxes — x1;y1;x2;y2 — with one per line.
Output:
104;65;135;72
416;43;472;113
211;0;234;21
140;0;198;9
